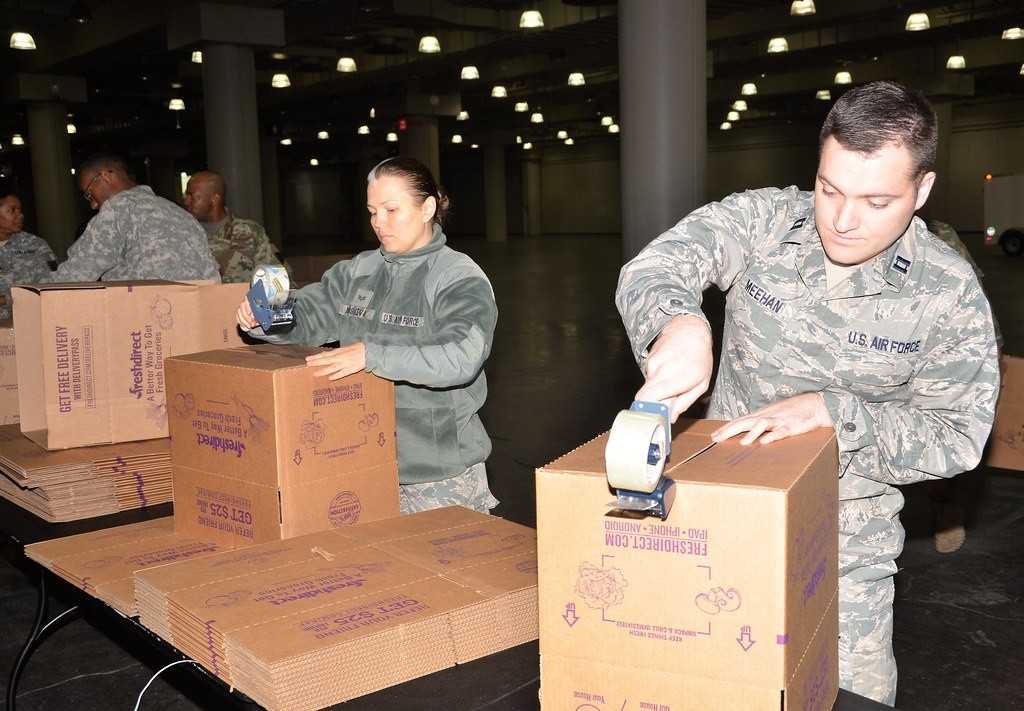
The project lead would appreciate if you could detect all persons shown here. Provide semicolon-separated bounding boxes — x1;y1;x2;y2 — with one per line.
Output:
615;80;1001;709
236;157;501;516
924;216;1004;552
185;171;299;290
0;190;58;318
38;152;222;285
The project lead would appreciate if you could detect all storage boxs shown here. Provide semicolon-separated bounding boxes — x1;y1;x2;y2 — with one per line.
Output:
163;345;401;556
9;278;267;452
536;416;839;711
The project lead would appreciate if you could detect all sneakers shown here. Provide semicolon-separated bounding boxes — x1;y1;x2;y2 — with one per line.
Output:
935;500;966;554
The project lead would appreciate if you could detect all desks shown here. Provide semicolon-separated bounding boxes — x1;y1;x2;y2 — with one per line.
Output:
0;497;893;711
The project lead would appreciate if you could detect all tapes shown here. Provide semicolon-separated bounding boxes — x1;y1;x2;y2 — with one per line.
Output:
604;409;667;494
249;263;291;305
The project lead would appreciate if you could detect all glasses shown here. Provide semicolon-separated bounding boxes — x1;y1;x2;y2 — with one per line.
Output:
81;170;117;200
